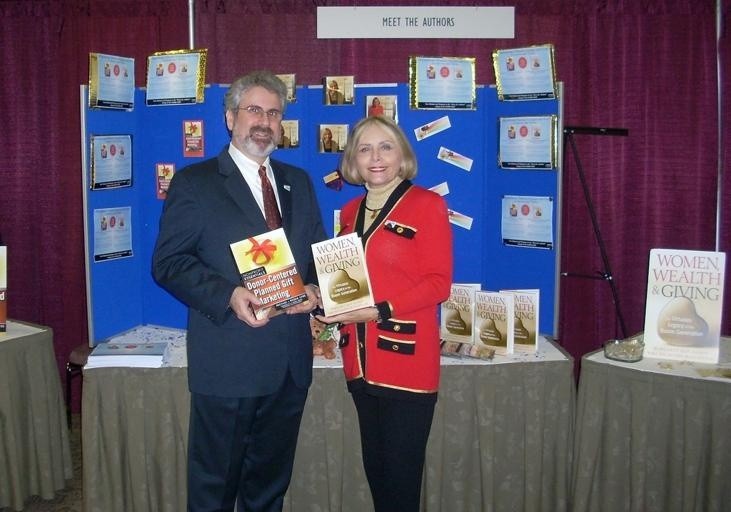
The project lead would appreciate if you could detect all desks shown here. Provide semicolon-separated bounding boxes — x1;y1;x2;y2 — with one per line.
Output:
0;318;75;512
575;330;731;512
80;323;576;512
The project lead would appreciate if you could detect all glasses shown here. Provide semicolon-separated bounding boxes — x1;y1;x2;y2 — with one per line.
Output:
236;105;284;121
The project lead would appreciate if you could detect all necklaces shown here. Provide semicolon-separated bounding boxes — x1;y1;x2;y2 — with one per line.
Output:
365;204;384;218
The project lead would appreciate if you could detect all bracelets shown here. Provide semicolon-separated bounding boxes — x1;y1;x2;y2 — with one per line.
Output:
373;300;393;321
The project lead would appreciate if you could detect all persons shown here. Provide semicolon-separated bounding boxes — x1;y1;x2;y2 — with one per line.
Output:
325;80;345;106
310;112;456;511
148;69;335;512
319;128;340;154
367;97;385;118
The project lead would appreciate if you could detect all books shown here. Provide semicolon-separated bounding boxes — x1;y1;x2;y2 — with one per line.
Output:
440;281;482;345
498;287;542;352
85;339;172;370
641;249;727;365
1;246;7;332
474;288;515;354
228;226;309;320
307;231;377;319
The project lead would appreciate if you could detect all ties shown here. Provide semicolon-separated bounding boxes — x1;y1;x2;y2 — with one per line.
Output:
258;165;282;230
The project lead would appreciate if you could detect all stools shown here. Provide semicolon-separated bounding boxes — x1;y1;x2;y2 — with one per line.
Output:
67;345;92;430
